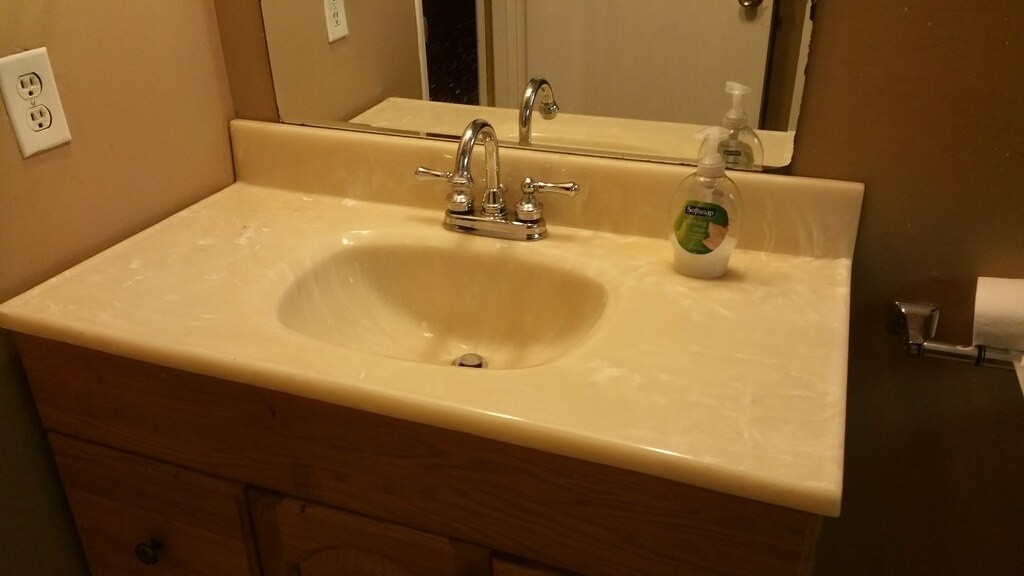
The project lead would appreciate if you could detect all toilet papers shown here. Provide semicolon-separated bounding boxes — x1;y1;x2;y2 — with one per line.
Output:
971;275;1024;395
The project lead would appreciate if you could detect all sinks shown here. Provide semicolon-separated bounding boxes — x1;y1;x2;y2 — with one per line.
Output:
505;132;650;157
272;230;623;374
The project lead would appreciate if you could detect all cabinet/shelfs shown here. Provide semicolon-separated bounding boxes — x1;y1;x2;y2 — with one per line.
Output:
10;330;817;575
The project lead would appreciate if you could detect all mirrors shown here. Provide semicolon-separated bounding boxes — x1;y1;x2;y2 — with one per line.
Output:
260;0;815;173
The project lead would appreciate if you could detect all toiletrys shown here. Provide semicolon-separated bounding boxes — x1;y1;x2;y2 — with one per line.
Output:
695;80;764;171
666;125;747;280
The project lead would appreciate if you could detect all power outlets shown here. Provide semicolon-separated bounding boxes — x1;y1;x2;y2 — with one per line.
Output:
324;0;349;43
0;46;73;159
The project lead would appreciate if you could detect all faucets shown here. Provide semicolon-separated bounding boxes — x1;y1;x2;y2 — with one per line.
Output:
447;118;508;239
518;76;560;148
